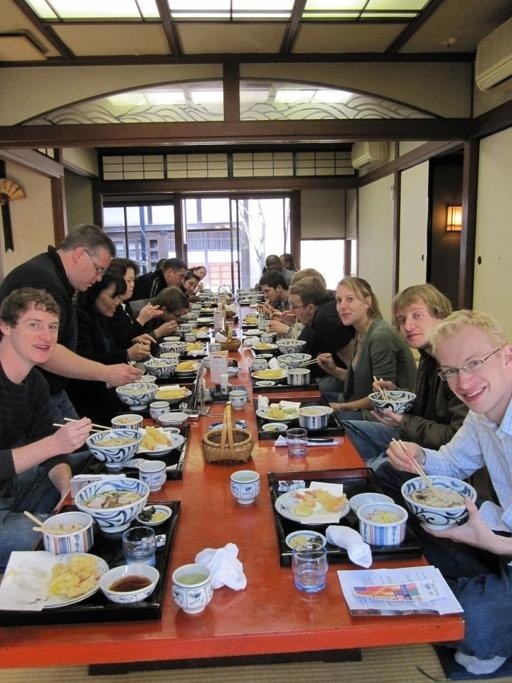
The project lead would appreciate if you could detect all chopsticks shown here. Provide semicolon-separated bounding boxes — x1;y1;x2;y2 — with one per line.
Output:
392;436;437;495
24;505;66;536
52;416;113;434
371;373;387;401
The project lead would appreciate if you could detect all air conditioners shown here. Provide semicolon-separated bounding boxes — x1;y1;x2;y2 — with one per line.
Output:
345;134;390;171
470;11;512;97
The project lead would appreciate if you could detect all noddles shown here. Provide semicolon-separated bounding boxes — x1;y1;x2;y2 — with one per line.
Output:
412;486;465;508
285;318;298;345
96;437;136;447
86;491;138;509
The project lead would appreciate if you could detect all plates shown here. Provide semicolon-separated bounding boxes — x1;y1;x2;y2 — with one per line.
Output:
137;505;172;526
44;552;109;608
275;488;350;525
286;529;325;550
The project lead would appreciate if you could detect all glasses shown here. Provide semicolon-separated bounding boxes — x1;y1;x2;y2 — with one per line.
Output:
84;248;107;277
289;303;310;313
437;347;501;382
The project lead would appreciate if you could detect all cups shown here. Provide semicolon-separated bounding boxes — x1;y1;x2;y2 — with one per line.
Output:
230;469;261;506
171;563;214;614
291;543;328;593
357;502;409;547
140;459;168;493
41;511;94;553
123;526;156;567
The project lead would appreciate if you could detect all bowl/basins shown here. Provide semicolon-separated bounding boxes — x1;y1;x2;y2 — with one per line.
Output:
400;475;478;531
74;478;150;534
368;391;418;421
350;490;395;514
100;563;160;604
86;285;335;469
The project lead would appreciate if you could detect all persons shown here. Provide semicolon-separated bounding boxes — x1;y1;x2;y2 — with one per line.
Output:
0;222;141;431
387;308;511;681
76;267;155;364
108;258;208;342
316;276;418;401
334;284;469;460
0;287;96;568
286;276;355;381
252;253;327;341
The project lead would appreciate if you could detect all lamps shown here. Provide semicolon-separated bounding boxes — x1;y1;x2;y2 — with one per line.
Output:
444;199;463;232
